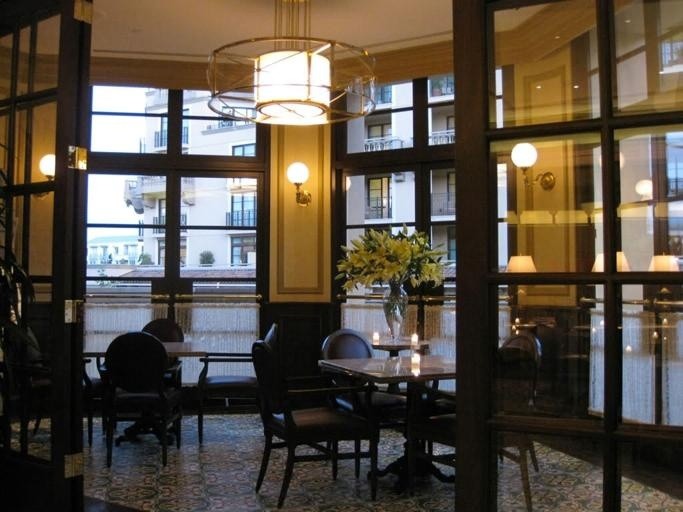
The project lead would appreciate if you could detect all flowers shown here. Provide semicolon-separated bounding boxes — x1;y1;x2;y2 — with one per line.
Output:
334;225;445;292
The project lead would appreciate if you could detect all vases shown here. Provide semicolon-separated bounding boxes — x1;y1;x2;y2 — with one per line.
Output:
382;279;409;340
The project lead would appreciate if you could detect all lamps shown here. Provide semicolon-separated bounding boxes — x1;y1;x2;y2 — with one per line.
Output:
285;161;313;205
205;1;378;127
28;152;58;202
588;251;631;287
504;255;536;324
647;255;679;310
511;142;554;191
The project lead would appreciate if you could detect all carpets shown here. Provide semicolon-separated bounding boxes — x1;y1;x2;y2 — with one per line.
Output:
0;415;681;512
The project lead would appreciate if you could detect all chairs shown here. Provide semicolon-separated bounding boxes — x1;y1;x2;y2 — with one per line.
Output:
255;319;456;508
495;328;541;511
82;318;209;468
5;320;94;457
